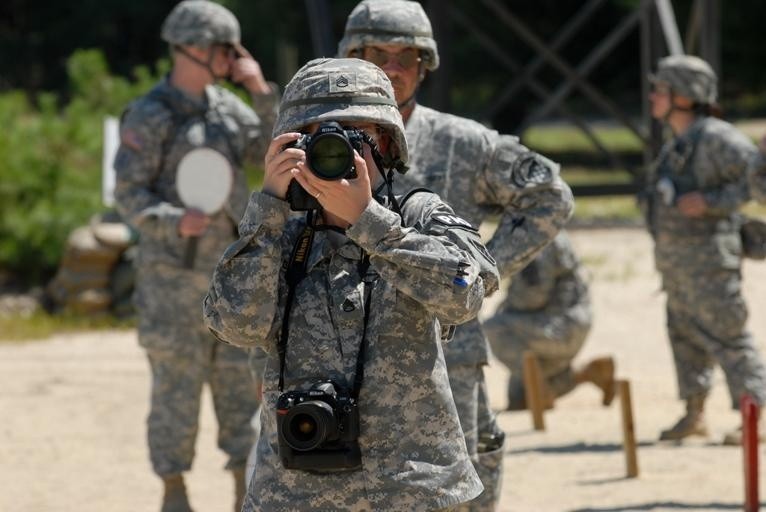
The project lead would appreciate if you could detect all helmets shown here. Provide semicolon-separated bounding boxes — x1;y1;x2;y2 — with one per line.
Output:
160;0;243;48
648;54;718;103
272;0;441;175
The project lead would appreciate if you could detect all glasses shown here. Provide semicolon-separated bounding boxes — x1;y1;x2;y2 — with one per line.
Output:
366;45;422;70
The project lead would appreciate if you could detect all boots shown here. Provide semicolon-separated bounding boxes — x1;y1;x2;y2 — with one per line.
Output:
580;358;616;404
660;394;708;440
723;410;766;444
160;472;191;512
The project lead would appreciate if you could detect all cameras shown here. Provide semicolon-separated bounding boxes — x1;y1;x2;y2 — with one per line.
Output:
282;122;364;211
275;379;361;473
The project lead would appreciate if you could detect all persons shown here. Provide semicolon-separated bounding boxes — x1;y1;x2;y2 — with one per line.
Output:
638;51;766;443
201;53;509;511
111;1;283;510
478;227;618;408
333;0;579;511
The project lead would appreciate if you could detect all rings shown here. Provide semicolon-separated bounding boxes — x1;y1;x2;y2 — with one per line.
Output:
314;191;322;200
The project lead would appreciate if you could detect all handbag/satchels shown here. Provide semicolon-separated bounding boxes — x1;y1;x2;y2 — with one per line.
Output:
741;221;765;257
